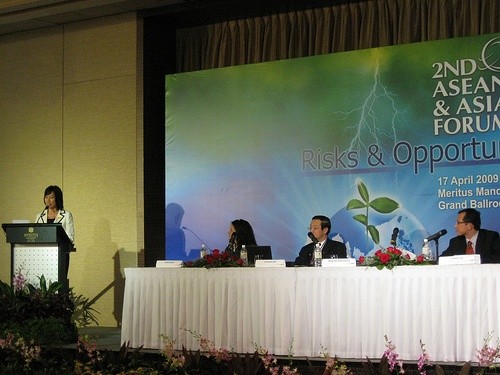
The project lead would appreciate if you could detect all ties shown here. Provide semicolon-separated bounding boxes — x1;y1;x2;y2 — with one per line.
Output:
466;241;474;254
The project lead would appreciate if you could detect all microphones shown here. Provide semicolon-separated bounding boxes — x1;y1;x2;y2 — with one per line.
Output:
225;232;238;251
37;205;50;223
390;228;399;244
424;229;447;242
308;232;321;247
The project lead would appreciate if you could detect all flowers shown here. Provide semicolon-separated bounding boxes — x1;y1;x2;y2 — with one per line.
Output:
184;247;244;270
358;247;427;269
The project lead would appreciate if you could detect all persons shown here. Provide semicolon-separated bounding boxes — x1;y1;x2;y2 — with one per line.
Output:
295;215;347;267
228;219;259;261
437;208;500;264
35;185;74;273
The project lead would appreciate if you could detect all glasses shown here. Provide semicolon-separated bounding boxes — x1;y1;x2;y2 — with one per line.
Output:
456;220;464;225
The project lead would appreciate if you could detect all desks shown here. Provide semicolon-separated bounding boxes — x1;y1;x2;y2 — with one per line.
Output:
120;263;499;362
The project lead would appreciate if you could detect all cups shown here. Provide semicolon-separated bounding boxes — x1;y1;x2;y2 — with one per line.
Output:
330;254;338;259
254;255;262;263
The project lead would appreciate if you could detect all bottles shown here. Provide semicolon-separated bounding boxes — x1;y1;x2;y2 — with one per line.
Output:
314;243;322;267
421;238;432;261
240;245;248;267
200;245;207;258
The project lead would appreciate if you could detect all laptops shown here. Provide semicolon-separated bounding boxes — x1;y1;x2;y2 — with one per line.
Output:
247;245;272;266
